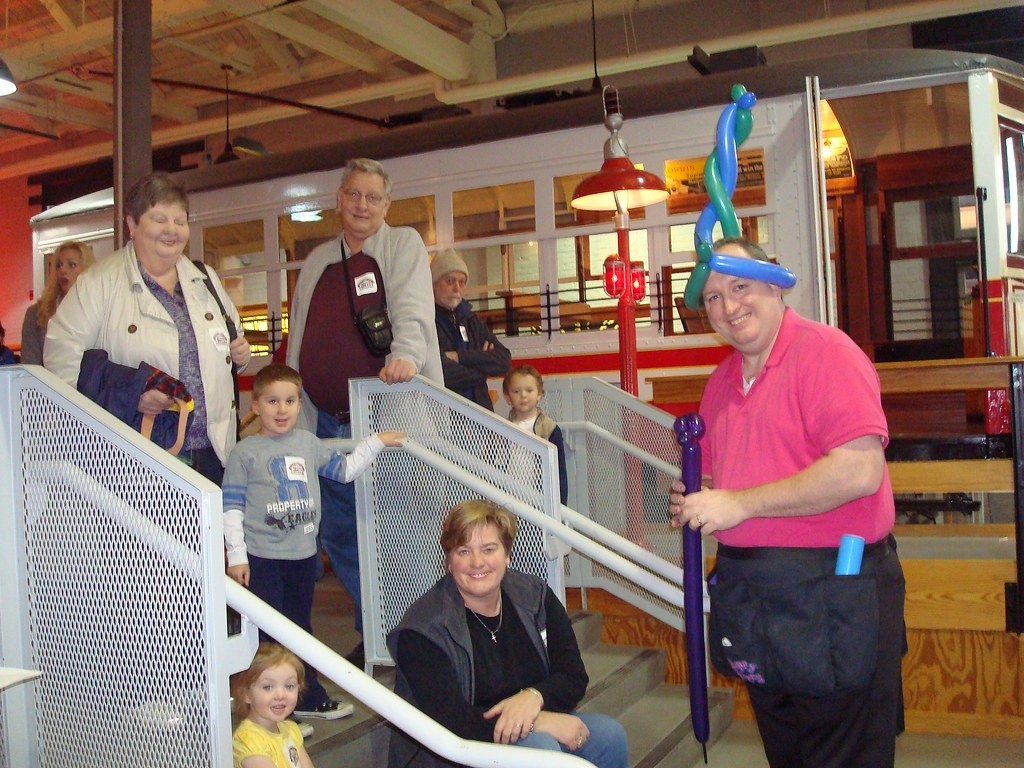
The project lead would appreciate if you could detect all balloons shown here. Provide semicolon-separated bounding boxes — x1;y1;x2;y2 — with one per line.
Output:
684;85;796;313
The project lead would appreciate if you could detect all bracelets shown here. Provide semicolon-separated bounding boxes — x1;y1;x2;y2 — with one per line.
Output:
520;688;544;704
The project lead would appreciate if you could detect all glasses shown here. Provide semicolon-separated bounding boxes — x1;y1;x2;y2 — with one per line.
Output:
340;186;386;206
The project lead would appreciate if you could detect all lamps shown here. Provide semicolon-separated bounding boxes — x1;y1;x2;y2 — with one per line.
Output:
588;0;609;95
232;136;268;156
214;66;241;162
568;84;670;210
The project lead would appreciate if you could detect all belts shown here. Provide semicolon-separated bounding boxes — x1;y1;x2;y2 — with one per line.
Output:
318;411;351;424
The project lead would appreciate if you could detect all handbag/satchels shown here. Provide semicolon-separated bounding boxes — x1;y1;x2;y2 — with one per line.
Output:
355;303;394;357
706;553;878;697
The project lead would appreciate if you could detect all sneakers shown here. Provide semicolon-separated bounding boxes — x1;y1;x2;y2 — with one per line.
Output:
293;698;354;721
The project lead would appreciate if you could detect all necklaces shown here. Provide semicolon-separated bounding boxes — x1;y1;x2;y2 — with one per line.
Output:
461;591;502;643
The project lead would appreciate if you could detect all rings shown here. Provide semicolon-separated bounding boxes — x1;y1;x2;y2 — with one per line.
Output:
514;723;522;728
576;736;583;744
696;514;703;526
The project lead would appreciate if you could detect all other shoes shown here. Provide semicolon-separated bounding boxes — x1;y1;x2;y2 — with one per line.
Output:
132;704;180;728
344;641;364;671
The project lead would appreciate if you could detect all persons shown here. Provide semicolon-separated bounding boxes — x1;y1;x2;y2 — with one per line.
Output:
221;362;406;739
20;240;96;366
286;159;446;676
386;499;629;768
493;366;569;583
430;247;512;464
229;641;315;768
669;238;908;768
43;172;251;728
0;321;21;365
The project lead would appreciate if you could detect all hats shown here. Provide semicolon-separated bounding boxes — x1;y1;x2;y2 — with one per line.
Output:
430;247;469;288
